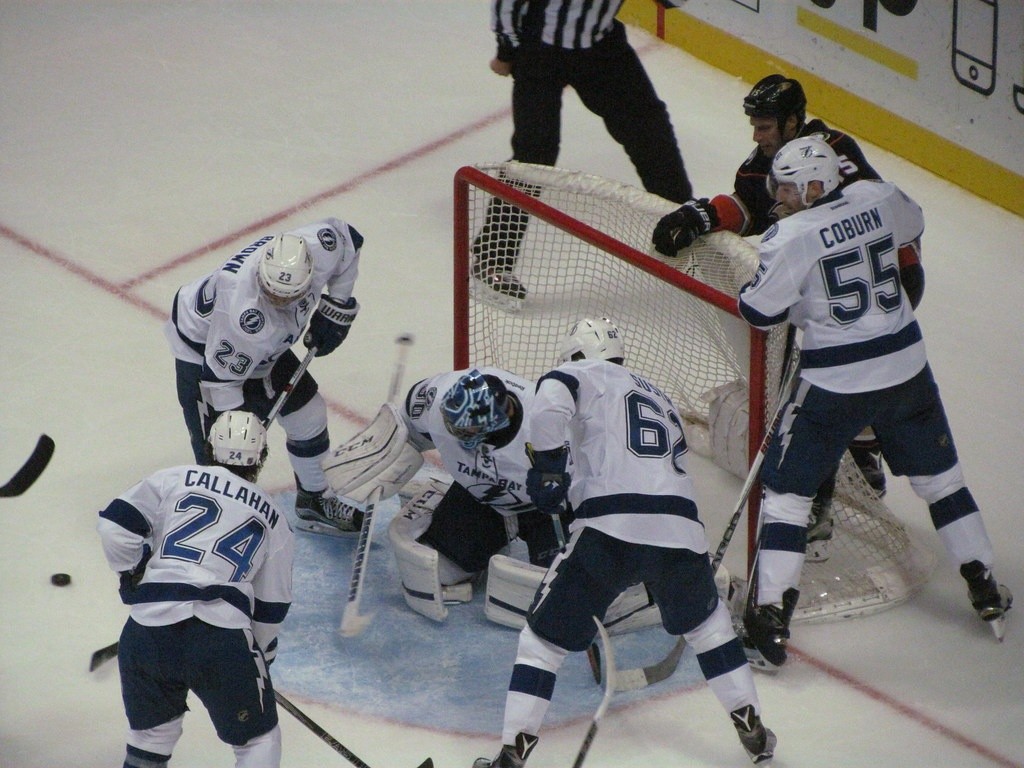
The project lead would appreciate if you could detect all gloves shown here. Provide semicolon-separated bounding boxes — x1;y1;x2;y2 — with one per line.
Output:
652;198;720;258
897;238;925;309
525;468;571;509
120;545;153;588
304;294;360;357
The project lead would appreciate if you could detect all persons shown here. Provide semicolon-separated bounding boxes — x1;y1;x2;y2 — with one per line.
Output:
466;314;778;767
92;409;295;768
652;75;926;498
166;216;370;539
385;363;664;636
736;137;1012;669
467;0;697;312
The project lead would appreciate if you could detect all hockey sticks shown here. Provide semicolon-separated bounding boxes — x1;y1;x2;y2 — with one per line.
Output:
574;616;617;767
270;690;433;767
261;345;318;431
1;434;55;499
337;492;380;639
589;358;804;687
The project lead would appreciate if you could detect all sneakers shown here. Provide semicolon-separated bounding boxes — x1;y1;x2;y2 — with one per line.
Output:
469;259;527;313
960;561;1013;643
738;588;800;671
849;438;887;498
293;471;370;540
732;705;777;768
473;732;539;768
804;519;833;562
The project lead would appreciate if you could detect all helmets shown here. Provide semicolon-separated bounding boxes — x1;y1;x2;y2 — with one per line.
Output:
557;317;626;362
440;370;510;450
254;233;314;312
766;136;844;209
743;75;807;119
205;411;269;473
